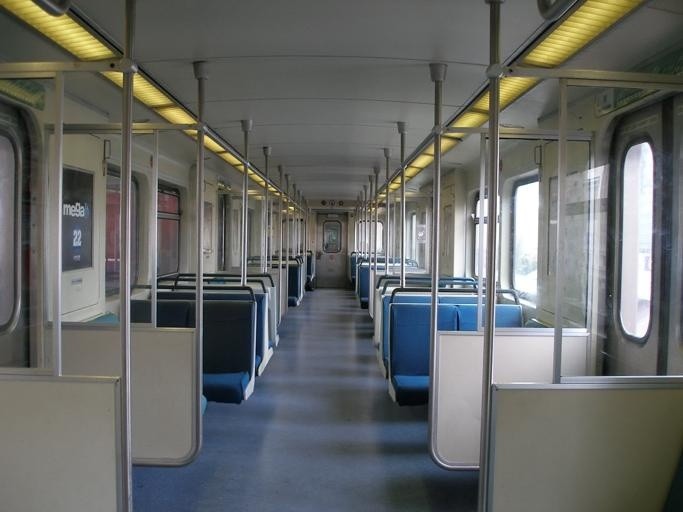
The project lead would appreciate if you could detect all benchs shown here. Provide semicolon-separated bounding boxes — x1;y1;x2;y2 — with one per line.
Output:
344;250;530;406
80;247;314;412
